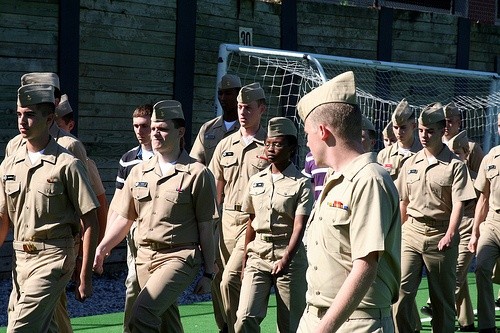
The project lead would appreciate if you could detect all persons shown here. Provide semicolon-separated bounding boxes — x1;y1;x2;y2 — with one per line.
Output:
295;71;402;333
187;74;243;333
111;104;154;333
5;73;90;333
446;129;478;333
233;117;315;333
0;83;102;333
55;93;111;265
360;98;423;184
466;112;500;333
207;82;273;333
420;102;487;320
92;100;221;333
303;152;330;200
392;102;477;333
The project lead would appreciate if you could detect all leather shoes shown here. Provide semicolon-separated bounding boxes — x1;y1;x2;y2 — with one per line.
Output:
494;298;500;307
420;304;433;317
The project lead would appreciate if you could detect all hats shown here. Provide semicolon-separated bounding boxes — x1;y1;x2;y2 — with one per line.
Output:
15;83;56;107
444;101;460;118
218;74;242;90
236;82;265;103
268;116;298;138
296;71;358;123
418;102;445;125
447;130;469;150
20;72;61;92
392;98;416;126
381;121;395;138
54;94;73;120
151;99;185;122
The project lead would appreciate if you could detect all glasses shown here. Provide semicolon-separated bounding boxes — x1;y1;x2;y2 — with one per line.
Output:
218;91;232;96
264;143;288;150
